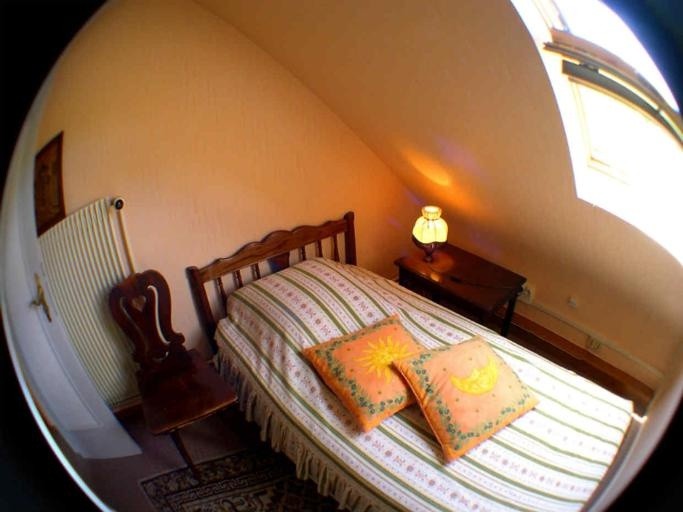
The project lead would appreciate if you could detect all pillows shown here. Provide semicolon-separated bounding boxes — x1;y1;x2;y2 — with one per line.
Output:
393;334;538;462
301;312;428;434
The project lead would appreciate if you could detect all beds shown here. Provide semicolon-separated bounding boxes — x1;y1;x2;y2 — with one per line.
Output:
185;211;638;512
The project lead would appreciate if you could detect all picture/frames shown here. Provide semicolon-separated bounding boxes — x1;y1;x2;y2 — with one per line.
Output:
33;131;66;236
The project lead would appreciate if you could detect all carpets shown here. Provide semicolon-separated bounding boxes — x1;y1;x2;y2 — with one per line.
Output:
138;444;326;512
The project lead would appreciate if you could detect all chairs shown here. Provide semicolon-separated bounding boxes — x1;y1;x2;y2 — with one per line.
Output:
109;269;238;484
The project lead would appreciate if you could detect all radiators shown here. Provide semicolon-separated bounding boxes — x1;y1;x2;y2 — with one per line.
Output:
37;195;143;414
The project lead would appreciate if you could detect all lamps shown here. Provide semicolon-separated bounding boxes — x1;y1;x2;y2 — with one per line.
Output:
411;204;450;261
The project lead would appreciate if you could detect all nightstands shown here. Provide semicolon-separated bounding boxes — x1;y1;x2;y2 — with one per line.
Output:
394;242;528;337
